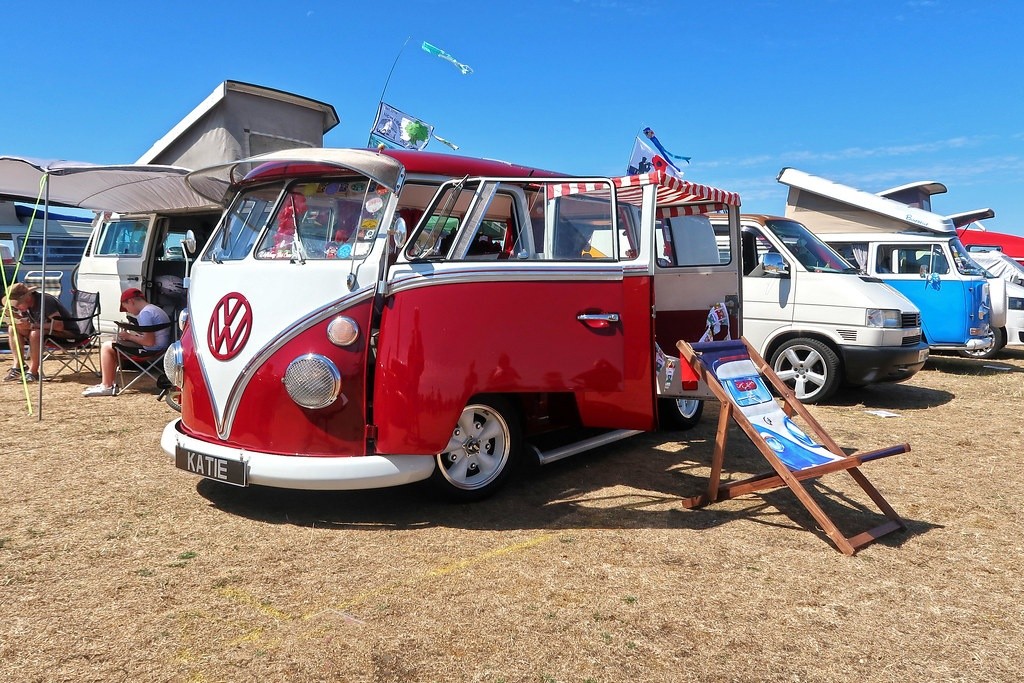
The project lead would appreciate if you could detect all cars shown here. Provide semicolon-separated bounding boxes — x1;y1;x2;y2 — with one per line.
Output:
956;229;1024;267
704;215;930;405
1000;281;1024;349
777;167;1006;352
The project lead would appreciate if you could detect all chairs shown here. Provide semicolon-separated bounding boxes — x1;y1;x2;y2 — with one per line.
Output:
22;285;102;376
110;313;182;395
673;335;911;558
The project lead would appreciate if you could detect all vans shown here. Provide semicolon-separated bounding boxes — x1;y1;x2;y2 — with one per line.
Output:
158;148;743;508
74;204;272;348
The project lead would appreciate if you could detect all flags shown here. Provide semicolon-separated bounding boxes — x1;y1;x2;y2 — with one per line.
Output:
626;137;684;180
371;101;434;150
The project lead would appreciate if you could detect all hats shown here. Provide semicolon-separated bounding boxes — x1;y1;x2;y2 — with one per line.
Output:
119;287;144;312
1;282;38;308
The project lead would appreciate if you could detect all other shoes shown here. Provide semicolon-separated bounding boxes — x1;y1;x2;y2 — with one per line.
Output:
82;382;119;396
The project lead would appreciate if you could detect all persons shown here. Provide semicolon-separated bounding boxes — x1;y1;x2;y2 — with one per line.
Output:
81;288;169;397
2;282;81;384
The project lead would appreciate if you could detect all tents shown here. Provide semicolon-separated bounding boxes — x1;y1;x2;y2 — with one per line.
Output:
0;156;238;421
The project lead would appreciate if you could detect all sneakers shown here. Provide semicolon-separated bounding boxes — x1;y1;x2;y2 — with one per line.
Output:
16;370;39;384
1;362;30;382
